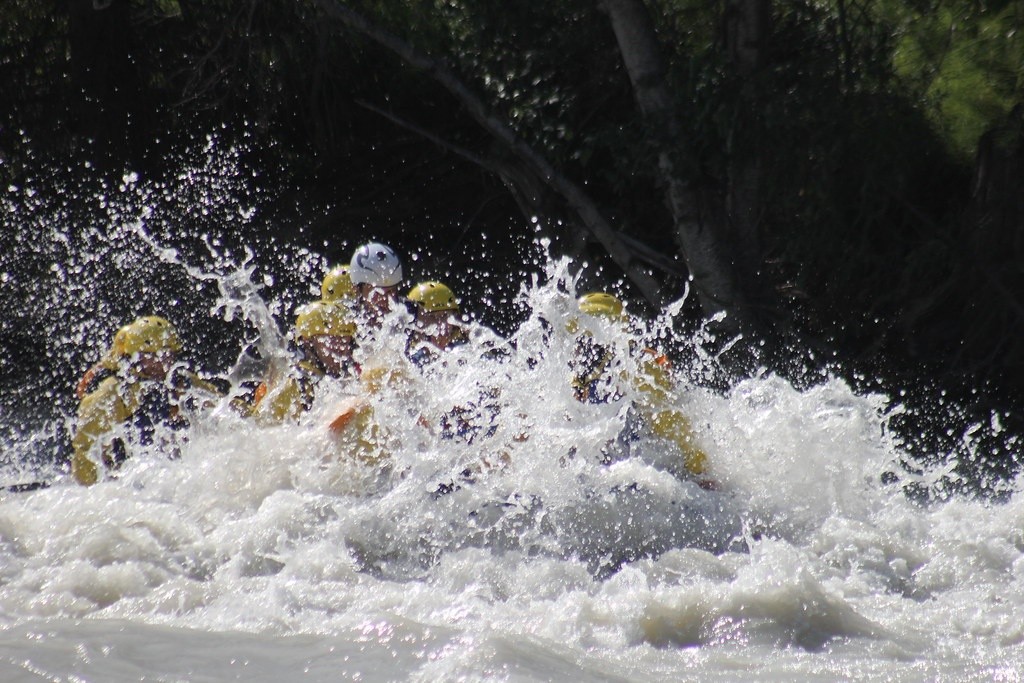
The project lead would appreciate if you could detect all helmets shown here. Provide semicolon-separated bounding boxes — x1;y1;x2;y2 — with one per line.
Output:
124;318;182;356
350;243;402;289
576;290;623;315
564;304;615;338
114;324;131;355
319;265;356;305
295;300;355;341
409;281;460;314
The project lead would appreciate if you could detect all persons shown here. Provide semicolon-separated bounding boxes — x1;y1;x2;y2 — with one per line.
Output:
69;237;723;513
